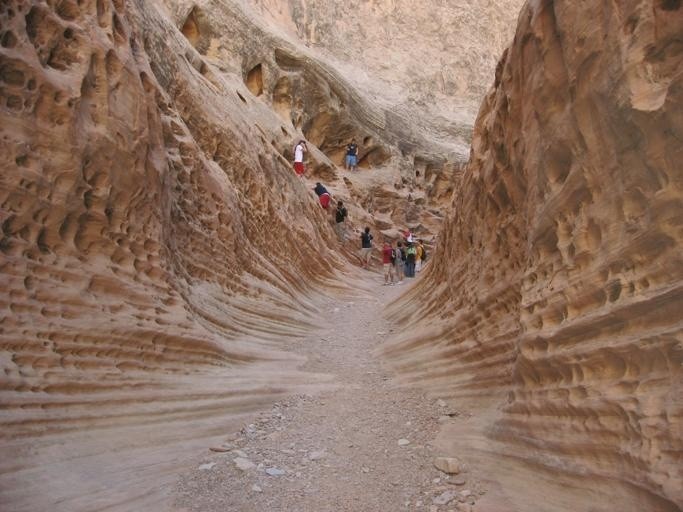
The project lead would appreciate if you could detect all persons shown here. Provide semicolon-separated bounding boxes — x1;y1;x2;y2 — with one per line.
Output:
382;226;424;286
358;227;373;269
312;182;330;208
344;139;358;171
333;201;348;241
294;140;307;175
